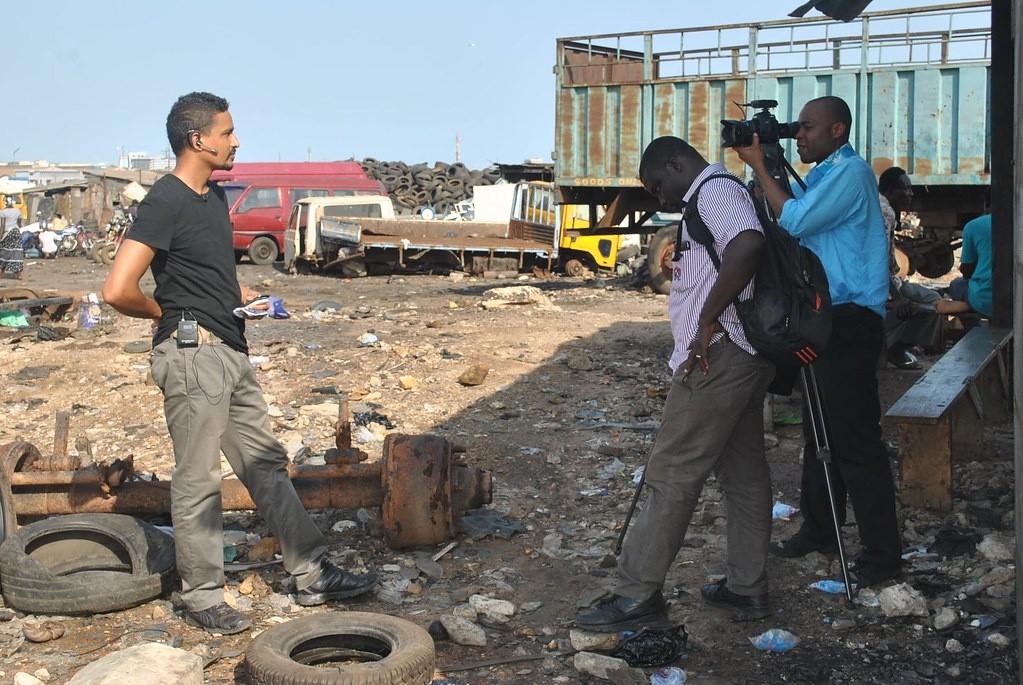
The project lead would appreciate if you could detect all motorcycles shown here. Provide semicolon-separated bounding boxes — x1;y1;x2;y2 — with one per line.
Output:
90;214;134;265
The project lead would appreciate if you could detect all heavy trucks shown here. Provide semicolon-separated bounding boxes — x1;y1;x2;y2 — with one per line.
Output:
551;0;993;295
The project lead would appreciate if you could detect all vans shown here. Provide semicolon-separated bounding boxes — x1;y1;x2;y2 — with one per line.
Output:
207;161;388;265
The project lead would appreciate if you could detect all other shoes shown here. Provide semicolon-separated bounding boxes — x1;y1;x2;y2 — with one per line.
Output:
842;555;900;586
888;342;918;369
771;527;837;558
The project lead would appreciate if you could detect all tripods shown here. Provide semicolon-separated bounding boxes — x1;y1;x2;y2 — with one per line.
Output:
615;145;857;610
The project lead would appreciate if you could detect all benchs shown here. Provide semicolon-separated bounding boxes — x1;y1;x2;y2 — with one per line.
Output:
882;326;1013;511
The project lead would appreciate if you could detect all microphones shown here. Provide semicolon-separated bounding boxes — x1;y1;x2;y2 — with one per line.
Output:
202;144;218;154
751;100;778;108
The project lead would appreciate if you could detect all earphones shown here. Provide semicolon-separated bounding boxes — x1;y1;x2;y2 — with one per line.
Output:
196;140;202;146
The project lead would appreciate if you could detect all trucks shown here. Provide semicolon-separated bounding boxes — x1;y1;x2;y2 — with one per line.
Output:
319;180;620;276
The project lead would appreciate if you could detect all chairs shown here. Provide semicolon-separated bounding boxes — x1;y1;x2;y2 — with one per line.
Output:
244;193;260;208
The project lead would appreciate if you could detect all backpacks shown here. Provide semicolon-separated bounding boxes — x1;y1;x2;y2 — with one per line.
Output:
683;174;832;369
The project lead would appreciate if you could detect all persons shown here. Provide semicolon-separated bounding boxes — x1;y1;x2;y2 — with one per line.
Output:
127;199;138;216
22;228;62;259
576;137;775;633
732;96;903;586
933;187;993;343
0;197;24;279
878;167;944;370
104;92;379;634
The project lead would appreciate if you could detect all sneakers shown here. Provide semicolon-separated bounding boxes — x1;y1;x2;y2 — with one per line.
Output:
172;602;252;635
298;561;378;606
701;577;770;610
576;591;669;633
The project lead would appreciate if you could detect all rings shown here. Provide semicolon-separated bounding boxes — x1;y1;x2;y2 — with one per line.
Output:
696;355;701;358
